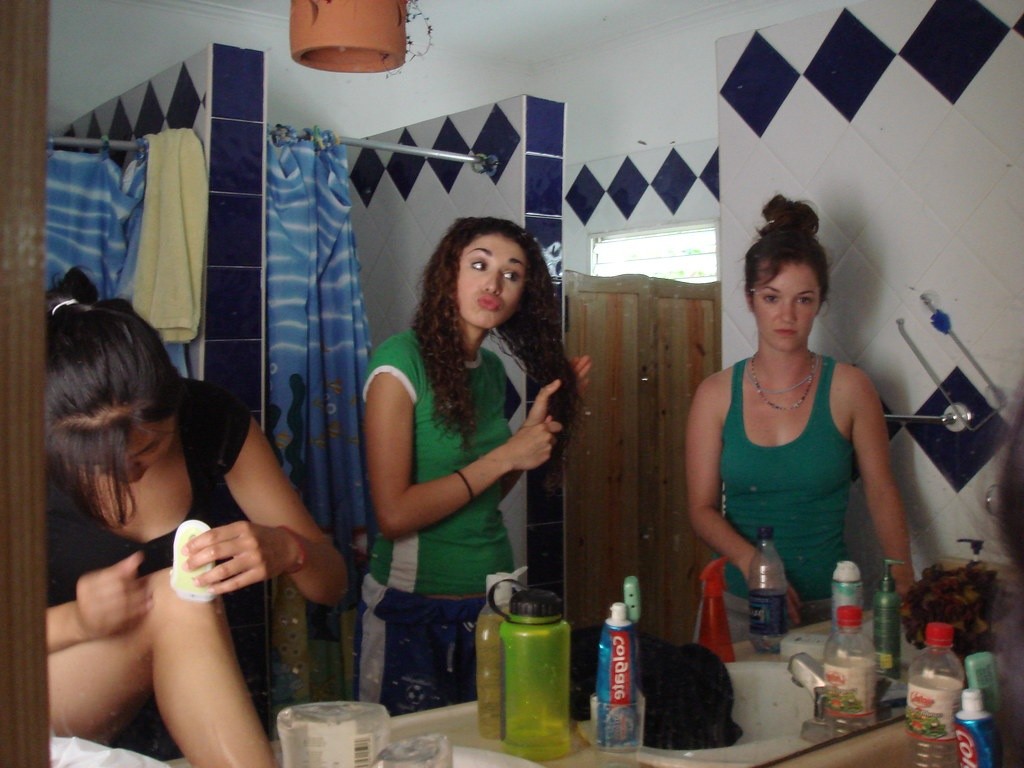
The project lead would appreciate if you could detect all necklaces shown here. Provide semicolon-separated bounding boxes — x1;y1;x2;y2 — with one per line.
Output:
744;349;818;410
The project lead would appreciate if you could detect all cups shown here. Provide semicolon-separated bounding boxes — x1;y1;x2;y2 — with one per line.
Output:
488;579;572;761
590;693;646;768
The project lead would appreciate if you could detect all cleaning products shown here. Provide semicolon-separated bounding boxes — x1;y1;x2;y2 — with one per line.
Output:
700;557;737;663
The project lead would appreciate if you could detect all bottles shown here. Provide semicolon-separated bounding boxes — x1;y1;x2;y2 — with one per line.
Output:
904;622;965;768
822;605;878;736
746;527;788;653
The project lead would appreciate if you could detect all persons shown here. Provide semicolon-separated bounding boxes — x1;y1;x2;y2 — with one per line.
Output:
47;266;348;768
362;215;592;717
684;194;914;645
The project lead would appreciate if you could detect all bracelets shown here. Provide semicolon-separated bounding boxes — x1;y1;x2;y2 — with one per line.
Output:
453;470;473;502
275;525;306;574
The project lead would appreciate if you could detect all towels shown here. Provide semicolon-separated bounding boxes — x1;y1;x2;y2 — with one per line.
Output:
131;128;208;345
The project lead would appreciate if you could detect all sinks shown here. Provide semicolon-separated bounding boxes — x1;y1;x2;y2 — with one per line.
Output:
585;661;909;768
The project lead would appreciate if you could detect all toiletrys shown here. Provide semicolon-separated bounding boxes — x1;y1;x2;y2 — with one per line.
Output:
593;603;638;752
872;558;909;681
955;687;1001;768
956;536;987;570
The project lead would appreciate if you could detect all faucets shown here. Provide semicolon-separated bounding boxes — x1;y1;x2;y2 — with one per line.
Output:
786;650;911;744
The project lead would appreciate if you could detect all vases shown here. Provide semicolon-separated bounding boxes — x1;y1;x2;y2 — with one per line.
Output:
290;1;407;73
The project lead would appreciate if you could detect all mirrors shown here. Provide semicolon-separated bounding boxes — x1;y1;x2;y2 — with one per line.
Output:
1;1;1024;768
